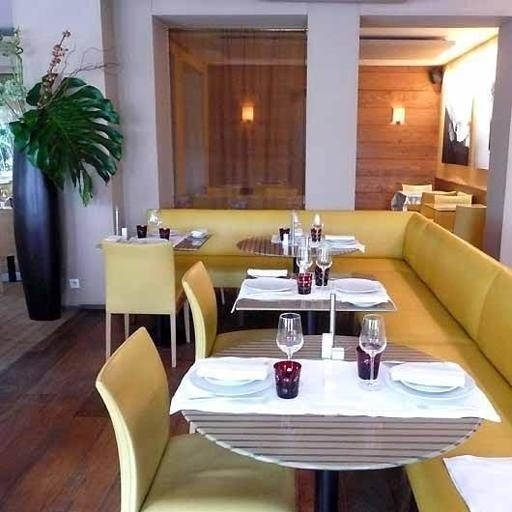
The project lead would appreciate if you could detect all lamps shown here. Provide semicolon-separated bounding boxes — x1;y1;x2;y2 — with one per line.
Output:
390;106;405;125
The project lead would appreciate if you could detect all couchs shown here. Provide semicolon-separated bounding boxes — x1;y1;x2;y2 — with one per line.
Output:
145;208;512;512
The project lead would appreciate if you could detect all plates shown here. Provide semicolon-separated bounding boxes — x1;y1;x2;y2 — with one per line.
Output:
384;371;476;402
243;278;295;293
334;278;380;295
202;374;256;387
391;380;465;393
189;363;275;399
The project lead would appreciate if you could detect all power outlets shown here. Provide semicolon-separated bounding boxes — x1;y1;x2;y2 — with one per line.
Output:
69;279;80;289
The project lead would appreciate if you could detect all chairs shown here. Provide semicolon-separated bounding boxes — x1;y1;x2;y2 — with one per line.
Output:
103;240;191;368
180;259;278;362
434;194;472;232
400;183;433;211
94;326;298;512
453;204;486;247
406;190;457;212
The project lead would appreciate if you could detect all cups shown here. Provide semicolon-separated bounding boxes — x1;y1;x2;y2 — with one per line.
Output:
279;227;290;242
276;312;304;361
145;208;160;238
135;224;149;241
158;226;172;239
295;244;315;273
359;313;387;393
313;241;333;292
274;360;301;399
297;272;313;295
356;343;382;380
314;263;330;286
311;228;322;242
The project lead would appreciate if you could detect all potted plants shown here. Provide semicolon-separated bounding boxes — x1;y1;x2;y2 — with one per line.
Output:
0;24;123;322
